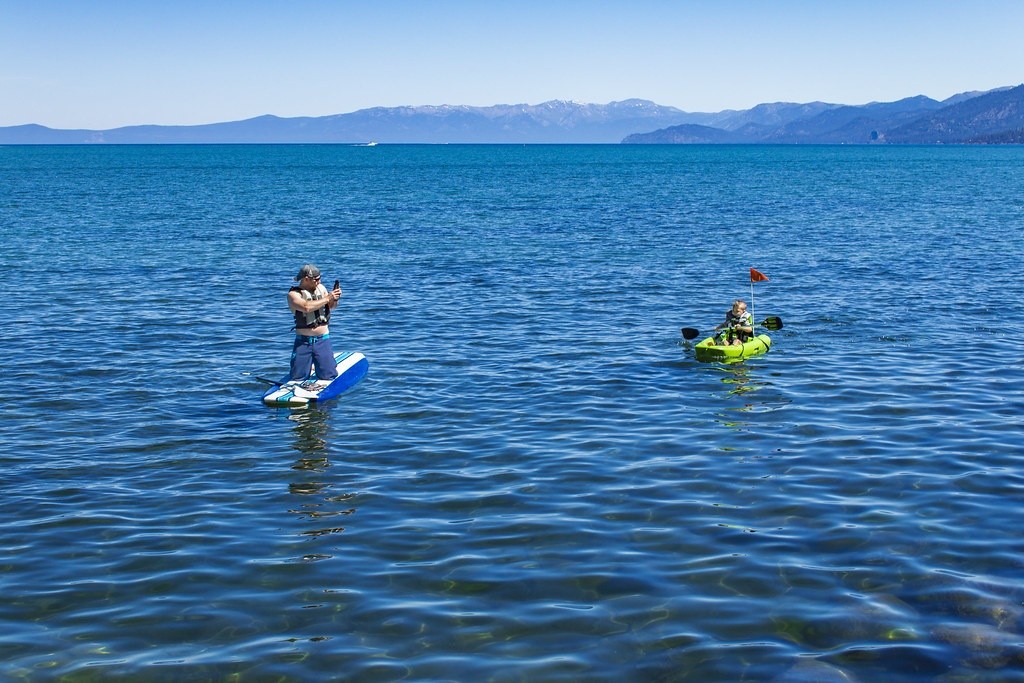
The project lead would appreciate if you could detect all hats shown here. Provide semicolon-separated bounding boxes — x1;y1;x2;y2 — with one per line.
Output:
293;264;320;282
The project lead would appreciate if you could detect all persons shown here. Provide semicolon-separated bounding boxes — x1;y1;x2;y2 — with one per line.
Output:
287;264;343;381
713;300;753;345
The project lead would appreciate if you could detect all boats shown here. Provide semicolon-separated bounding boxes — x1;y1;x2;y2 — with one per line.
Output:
262;351;369;407
695;334;771;364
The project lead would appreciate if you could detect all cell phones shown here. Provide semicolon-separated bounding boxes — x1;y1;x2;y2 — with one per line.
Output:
334;280;340;290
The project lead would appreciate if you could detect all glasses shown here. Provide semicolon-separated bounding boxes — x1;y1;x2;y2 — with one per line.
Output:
311;275;321;282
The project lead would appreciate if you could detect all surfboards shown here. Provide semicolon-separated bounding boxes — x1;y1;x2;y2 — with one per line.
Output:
262;350;369;403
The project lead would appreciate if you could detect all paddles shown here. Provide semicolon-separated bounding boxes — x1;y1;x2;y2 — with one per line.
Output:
681;316;783;340
241;371;319;399
